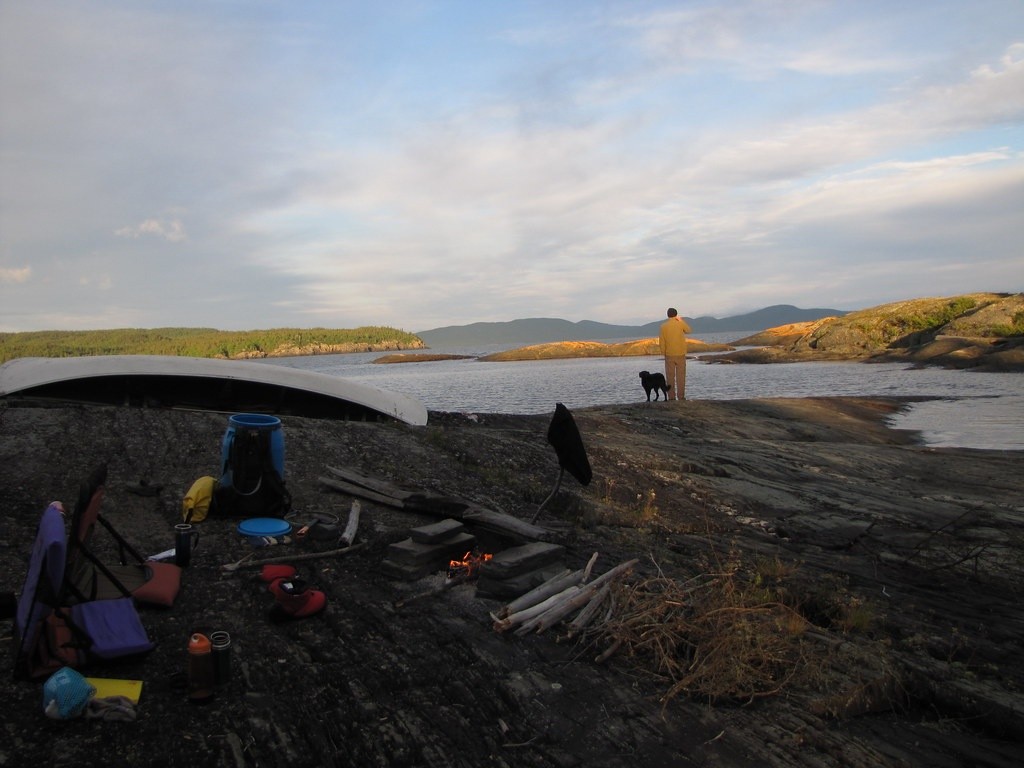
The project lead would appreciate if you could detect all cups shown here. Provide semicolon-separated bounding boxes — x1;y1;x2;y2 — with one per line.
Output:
175;523;199;568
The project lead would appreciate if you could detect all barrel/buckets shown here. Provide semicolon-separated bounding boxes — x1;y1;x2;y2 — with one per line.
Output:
221;413;285;481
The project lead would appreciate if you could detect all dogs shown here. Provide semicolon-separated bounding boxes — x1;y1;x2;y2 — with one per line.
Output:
639;371;672;402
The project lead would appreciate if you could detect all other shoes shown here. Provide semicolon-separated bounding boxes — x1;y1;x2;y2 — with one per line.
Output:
678;396;686;401
668;398;676;401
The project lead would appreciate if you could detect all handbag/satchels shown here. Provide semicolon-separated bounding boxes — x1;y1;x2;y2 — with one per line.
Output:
132;561;184;607
71;596;151;658
183;476;217;523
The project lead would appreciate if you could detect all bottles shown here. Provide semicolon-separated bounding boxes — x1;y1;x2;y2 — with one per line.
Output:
188;633;216;691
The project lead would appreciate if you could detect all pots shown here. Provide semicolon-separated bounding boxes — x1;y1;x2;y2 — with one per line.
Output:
283;509;339;538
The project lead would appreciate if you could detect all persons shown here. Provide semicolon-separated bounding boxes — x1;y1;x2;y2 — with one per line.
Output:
659;308;691;400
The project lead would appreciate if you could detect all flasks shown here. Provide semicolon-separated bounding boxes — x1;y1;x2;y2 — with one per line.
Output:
210;631;234;682
